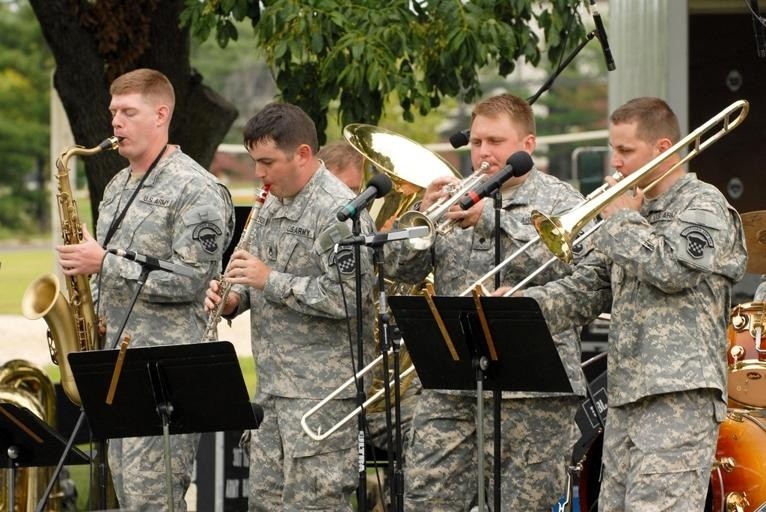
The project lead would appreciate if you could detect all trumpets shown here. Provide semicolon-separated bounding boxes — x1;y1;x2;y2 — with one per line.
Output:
398;159;494;251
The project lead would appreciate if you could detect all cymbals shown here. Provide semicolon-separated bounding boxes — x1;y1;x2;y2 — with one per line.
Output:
740;211;766;275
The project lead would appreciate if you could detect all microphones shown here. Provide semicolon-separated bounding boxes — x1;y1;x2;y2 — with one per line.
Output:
109;248;194;279
592;11;616;72
449;129;471;149
337;174;392;222
458;151;534;208
339;226;429;245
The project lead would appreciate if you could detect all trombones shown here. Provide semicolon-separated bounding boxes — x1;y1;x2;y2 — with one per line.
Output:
298;100;750;441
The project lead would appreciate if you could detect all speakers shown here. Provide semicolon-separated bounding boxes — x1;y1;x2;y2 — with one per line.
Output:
183;429;253;511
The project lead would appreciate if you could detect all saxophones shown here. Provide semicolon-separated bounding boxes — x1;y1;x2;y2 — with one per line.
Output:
22;137;123;407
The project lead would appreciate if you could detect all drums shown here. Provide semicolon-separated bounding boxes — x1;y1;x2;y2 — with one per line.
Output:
709;410;765;512
727;302;765;410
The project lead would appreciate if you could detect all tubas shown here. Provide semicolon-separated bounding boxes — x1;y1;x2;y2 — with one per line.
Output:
0;360;65;511
343;122;466;413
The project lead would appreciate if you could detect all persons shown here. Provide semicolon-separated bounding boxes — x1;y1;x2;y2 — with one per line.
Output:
204;100;384;512
315;141;423;512
376;94;601;511
52;68;239;512
486;94;750;512
